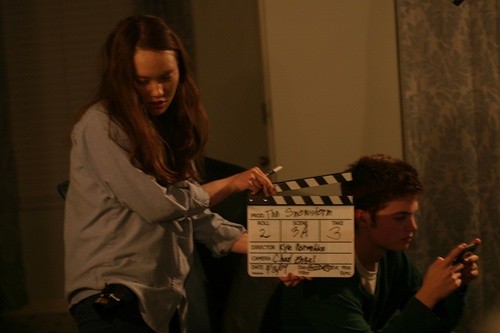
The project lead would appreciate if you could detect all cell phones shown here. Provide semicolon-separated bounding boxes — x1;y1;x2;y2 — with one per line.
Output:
452;243;476;275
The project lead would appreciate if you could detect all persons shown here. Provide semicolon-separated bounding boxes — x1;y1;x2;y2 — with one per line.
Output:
272;154;480;333
62;14;312;333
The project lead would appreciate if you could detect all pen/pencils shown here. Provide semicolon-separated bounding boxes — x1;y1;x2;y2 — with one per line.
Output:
265;166;283;177
